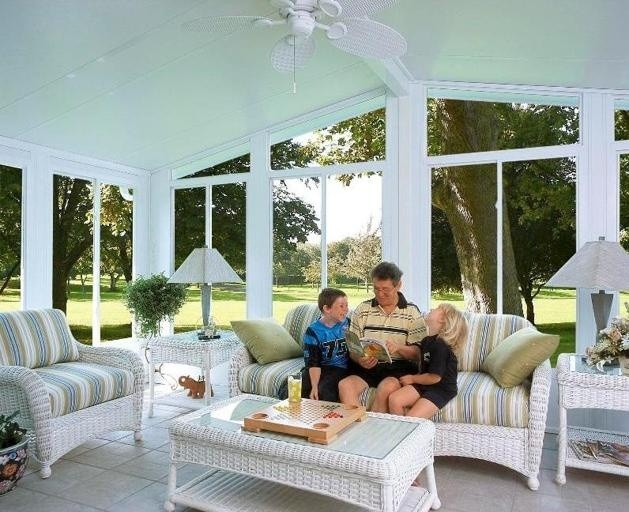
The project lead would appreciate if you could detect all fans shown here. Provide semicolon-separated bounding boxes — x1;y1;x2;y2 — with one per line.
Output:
183;0;409;76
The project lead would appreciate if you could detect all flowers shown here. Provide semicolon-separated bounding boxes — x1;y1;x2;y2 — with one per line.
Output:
585;302;629;373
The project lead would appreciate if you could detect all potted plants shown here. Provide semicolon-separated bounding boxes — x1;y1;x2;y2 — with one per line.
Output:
0;408;32;497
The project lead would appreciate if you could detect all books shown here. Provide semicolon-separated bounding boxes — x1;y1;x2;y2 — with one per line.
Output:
568;437;629;466
344;329;392;364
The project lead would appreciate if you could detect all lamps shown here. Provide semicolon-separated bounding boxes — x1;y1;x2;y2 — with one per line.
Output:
540;235;629;367
166;244;246;327
285;11;317;94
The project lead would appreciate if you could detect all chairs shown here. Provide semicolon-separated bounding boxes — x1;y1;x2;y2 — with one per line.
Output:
1;307;146;480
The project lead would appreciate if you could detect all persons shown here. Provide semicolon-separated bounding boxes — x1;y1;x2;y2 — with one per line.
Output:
339;262;427;414
279;287;352;402
388;302;469;486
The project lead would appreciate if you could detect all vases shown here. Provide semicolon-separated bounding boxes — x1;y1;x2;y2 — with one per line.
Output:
619;356;629;376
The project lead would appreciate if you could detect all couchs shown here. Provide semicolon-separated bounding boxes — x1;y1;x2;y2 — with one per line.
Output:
227;301;554;493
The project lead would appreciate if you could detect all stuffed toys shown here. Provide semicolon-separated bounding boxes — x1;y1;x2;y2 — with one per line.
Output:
179;376;214;399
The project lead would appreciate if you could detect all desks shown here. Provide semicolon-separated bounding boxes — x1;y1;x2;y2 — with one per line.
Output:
556;352;629;486
147;330;242;421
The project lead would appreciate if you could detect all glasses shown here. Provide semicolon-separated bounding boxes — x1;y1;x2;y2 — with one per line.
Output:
372;285;397;295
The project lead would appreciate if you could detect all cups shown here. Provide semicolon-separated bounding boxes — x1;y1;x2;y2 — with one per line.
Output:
287;371;303;402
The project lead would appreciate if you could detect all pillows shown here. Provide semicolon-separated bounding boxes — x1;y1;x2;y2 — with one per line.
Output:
229;317;304;366
480;327;561;391
